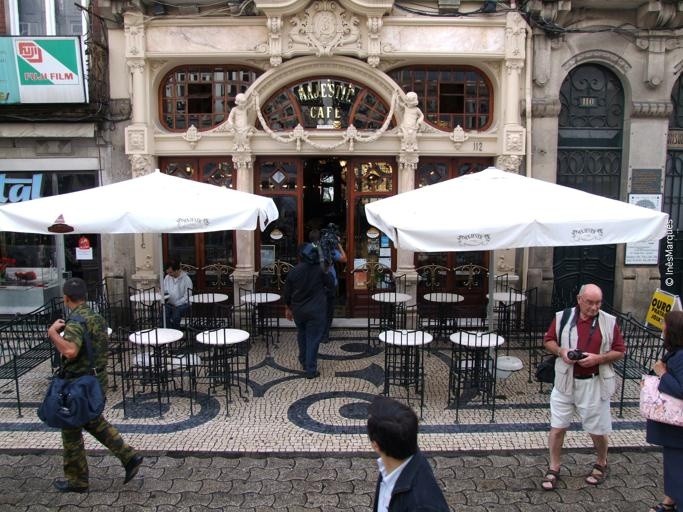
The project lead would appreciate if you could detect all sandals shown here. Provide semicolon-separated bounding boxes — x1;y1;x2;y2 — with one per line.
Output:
541;467;560;491
585;463;610;484
650;502;676;512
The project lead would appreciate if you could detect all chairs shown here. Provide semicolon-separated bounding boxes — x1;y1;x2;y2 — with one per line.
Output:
44;285;279;417
366;272;553;425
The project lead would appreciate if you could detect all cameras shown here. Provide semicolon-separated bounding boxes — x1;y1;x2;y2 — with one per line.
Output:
567;348;585;360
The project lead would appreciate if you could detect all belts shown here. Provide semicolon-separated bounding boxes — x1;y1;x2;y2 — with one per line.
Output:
573;372;599;379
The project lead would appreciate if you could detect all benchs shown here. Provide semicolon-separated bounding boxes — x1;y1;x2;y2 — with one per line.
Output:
607;311;667;419
0;306;56;418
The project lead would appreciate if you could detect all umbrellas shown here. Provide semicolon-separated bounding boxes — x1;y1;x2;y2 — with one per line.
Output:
365;168;669;371
0;168;279;356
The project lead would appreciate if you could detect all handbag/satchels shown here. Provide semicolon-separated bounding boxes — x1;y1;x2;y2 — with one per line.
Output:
535;354;554;381
639;374;683;427
37;373;106;429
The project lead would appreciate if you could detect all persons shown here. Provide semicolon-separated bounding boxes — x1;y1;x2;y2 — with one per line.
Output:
282;243;336;380
309;229;348;342
541;284;625;489
49;278;143;493
367;394;450;512
646;311;683;511
162;260;193;330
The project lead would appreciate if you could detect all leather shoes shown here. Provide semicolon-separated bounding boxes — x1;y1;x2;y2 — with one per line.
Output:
123;452;143;484
54;480;87;493
